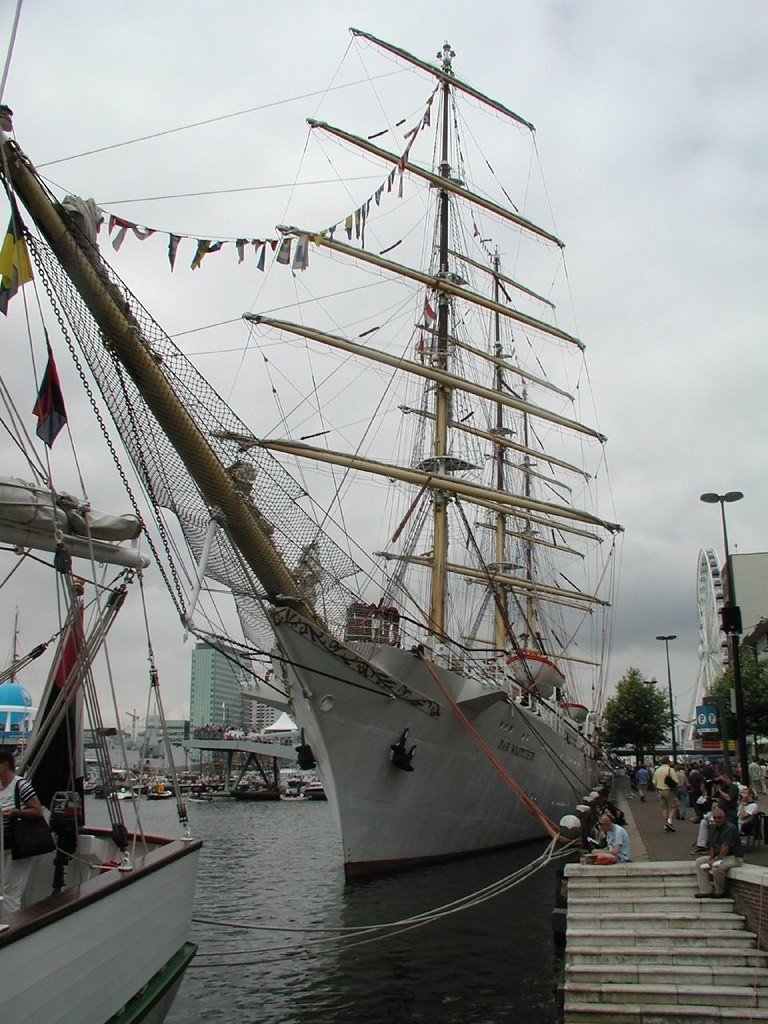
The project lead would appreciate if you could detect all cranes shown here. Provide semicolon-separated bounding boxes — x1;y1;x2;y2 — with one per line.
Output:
124;708;140;738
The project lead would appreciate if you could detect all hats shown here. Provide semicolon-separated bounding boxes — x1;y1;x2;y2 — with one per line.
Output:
705;760;710;765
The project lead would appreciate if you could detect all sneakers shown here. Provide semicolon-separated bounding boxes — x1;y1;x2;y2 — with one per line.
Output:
663;822;677;833
688;846;706;854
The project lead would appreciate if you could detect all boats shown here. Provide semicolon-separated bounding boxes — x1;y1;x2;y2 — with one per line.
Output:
83;768;328;802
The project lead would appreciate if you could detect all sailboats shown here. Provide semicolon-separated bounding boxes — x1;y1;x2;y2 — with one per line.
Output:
0;25;625;877
0;376;204;1024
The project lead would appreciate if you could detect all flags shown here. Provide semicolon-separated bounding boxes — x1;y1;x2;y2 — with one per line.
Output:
423;295;436;328
110;94;434;270
0;193;34;316
33;327;67;449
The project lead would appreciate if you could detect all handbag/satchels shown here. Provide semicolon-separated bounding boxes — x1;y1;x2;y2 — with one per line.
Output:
664;769;677;788
8;780;58;861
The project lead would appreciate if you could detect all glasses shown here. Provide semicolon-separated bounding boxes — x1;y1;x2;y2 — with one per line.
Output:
598;821;606;825
713;815;721;819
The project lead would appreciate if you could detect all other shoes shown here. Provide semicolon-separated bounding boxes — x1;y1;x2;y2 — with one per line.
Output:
676;815;685;820
760;791;766;795
640;796;647;802
695;893;726;898
755;796;758;800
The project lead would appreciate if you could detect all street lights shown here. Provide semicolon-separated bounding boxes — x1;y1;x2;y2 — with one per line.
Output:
656;635;677;765
700;490;753;780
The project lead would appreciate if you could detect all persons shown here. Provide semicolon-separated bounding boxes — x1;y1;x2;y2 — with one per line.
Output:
636;755;767;899
591;795;630;863
0;750;43;917
